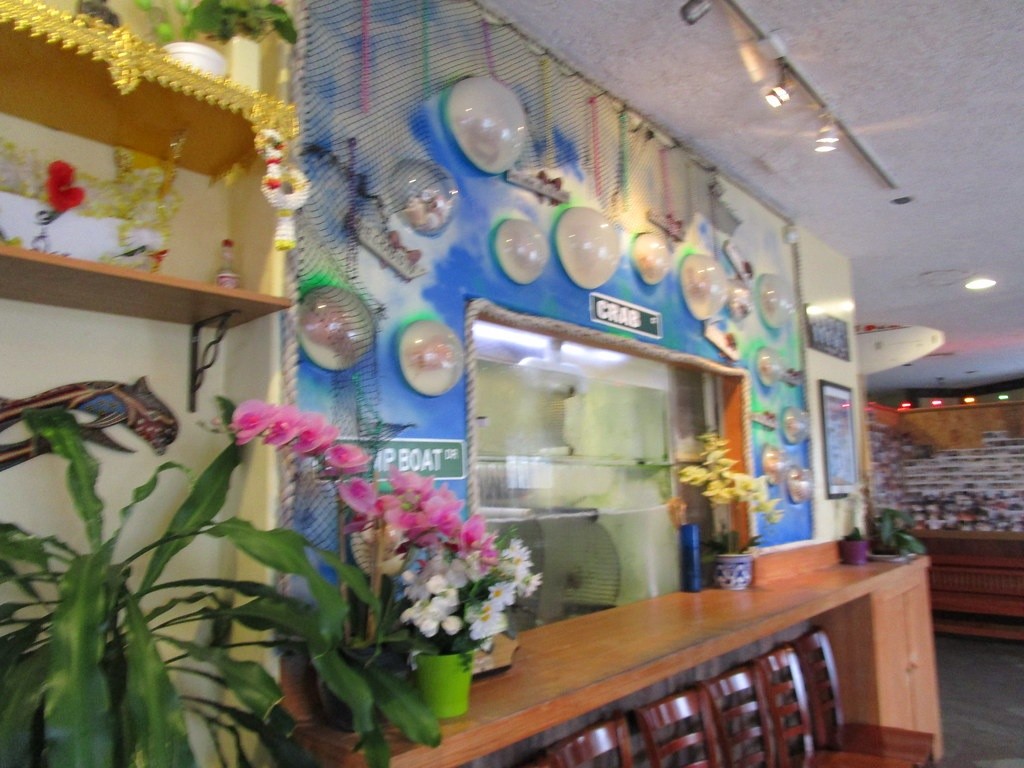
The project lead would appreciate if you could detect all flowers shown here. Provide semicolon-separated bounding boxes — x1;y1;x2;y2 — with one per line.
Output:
350;526;538;646
678;427;785;556
191;398;502;652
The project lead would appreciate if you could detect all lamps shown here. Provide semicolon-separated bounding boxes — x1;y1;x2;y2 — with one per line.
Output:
766;90;783;108
773;79;798;103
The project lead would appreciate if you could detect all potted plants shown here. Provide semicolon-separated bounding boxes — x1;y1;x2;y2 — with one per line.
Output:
870;508;927;561
843;527;869;565
135;0;300;84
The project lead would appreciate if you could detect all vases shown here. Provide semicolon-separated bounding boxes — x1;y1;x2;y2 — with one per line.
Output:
414;649;473;719
714;553;754;592
314;653;415;735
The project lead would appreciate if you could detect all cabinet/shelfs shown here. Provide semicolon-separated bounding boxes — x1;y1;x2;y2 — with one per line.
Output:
2;0;298;413
277;539;941;768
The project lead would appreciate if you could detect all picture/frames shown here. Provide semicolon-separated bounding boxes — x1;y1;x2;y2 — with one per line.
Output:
817;379;859;501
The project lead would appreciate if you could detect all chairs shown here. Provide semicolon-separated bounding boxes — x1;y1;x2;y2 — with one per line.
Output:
693;661;776;768
520;708;633;768
635;688;726;768
792;627;935;767
754;642;913;767
